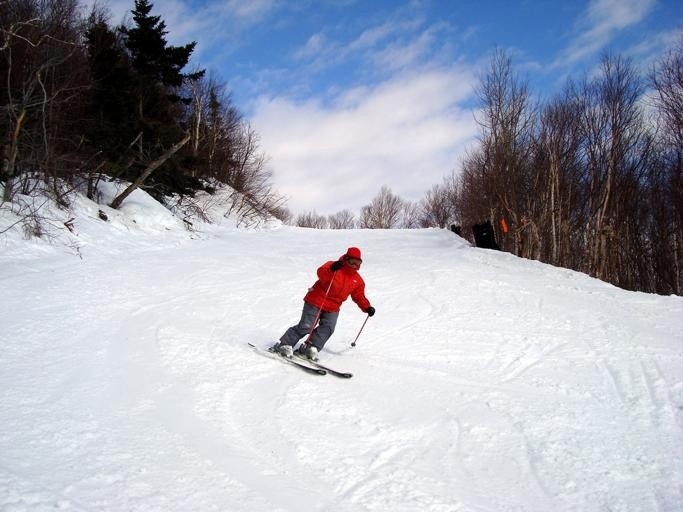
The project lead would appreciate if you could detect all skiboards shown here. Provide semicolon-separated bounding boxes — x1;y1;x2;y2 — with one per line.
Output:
249;343;352;378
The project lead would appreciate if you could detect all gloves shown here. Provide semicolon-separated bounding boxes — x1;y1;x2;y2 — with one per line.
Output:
366;307;375;316
330;261;342;270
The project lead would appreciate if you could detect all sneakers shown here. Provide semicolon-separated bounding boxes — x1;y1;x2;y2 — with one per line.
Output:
273;341;320;362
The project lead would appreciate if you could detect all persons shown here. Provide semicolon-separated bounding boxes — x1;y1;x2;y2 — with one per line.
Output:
275;247;376;361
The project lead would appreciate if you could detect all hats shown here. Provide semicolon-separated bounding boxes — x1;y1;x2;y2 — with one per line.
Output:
345;247;361;259
